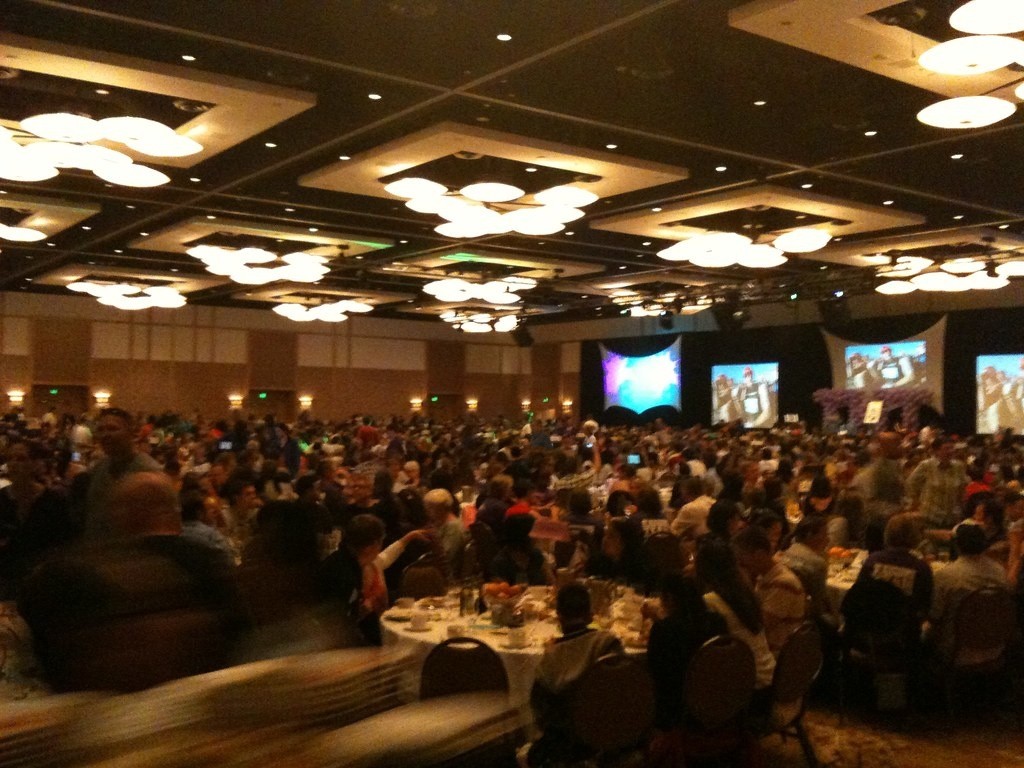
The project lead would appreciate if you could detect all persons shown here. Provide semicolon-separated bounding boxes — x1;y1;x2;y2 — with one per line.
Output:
357;416;379;447
849;346;922;390
0;406;1024;768
980;357;1024;435
712;368;778;428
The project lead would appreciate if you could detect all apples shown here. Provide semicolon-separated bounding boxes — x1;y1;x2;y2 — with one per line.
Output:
485;581;522;599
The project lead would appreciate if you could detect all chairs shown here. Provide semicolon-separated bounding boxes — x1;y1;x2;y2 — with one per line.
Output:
402;531;1014;768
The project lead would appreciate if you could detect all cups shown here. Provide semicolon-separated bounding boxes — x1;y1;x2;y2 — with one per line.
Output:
507;629;529;642
447;624;464;638
409;611;427;627
395;596;414;609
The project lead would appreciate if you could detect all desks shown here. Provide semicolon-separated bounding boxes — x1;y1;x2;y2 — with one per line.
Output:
825;548;868;608
383;585;648;739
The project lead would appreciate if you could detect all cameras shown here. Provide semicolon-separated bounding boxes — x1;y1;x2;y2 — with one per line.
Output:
218;441;235;450
70;452;81;463
586;443;593;448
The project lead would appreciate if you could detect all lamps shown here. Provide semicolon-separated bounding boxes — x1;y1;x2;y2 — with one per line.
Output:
0;0;1024;333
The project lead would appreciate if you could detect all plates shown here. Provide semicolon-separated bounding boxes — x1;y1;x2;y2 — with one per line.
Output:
500;642;532;650
403;623;432;632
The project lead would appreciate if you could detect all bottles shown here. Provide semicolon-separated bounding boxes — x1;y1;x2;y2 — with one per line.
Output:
460;577;474;616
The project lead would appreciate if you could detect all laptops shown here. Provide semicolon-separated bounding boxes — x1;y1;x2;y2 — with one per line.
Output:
626;454;642;465
